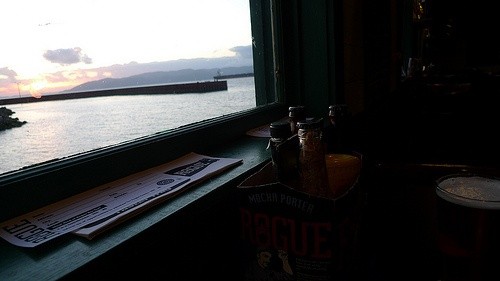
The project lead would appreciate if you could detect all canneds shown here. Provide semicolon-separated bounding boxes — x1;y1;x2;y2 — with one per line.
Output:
295;121;321;179
288;106;305;144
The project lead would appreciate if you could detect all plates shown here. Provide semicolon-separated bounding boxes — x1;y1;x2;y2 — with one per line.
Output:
435;173;500;209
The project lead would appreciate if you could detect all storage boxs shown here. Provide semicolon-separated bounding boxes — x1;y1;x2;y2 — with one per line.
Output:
237;151;362;281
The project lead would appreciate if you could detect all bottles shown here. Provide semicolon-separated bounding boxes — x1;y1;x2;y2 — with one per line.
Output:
324;103;351;154
288;106;305;133
270;122;295;186
296;121;328;200
